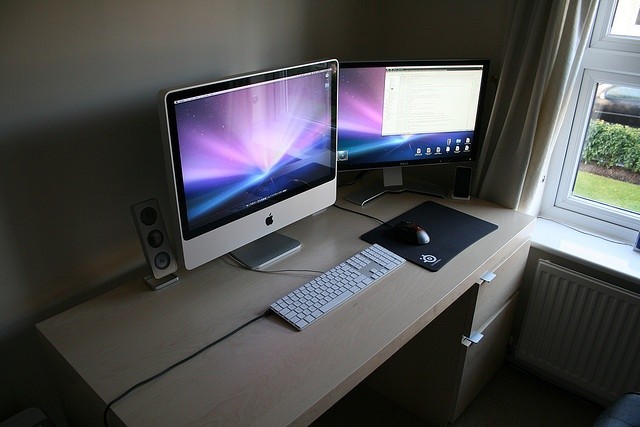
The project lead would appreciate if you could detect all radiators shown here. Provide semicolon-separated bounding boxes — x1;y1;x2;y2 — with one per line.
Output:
514;258;640;410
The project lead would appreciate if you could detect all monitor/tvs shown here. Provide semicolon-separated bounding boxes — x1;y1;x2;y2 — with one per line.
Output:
338;57;492;207
163;56;343;272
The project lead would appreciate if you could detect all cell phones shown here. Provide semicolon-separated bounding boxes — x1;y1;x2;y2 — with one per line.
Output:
450;163;474;201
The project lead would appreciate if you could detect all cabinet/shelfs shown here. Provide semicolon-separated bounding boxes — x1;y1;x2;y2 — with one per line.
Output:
362;235;534;427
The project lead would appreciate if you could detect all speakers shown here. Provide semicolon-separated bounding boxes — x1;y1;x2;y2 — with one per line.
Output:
128;197;179;281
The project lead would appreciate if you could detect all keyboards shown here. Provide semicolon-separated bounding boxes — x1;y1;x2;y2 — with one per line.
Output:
267;240;407;333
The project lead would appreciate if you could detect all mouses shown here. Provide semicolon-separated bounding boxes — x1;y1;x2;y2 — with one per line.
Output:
391;219;431;246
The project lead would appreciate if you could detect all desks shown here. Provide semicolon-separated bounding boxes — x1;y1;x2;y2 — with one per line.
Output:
23;170;537;427
